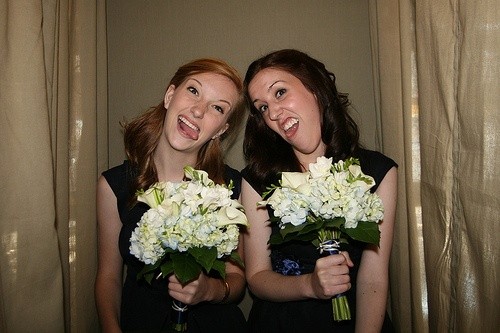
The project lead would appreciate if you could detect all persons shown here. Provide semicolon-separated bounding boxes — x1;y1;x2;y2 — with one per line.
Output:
241;50;399;333
94;59;245;333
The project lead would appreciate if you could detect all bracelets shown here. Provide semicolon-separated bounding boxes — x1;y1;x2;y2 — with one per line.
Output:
211;279;231;304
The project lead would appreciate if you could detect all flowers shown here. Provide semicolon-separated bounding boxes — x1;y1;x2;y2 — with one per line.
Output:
257;156;384;321
129;166;250;332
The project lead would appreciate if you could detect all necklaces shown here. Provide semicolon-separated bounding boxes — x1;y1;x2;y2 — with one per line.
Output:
299;163;307;171
